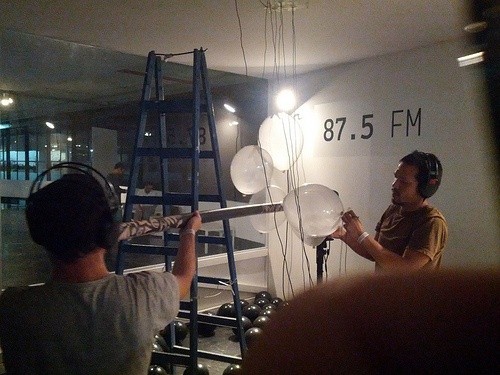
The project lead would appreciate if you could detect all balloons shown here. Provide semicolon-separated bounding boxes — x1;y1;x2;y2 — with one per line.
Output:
231;113;344;248
147;290;292;375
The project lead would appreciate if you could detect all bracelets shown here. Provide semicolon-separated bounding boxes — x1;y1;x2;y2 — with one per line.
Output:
357;232;370;246
179;228;195;238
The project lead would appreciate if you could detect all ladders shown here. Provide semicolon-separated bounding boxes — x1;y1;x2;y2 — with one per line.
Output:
115;47;248;373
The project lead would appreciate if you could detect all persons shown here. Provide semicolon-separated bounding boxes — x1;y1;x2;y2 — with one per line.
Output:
238;268;500;375
0;162;202;375
327;150;449;275
139;182;157;219
106;163;129;224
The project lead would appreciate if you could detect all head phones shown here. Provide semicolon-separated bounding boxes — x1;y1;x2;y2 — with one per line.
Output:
26;162;122;249
420;151;440;198
